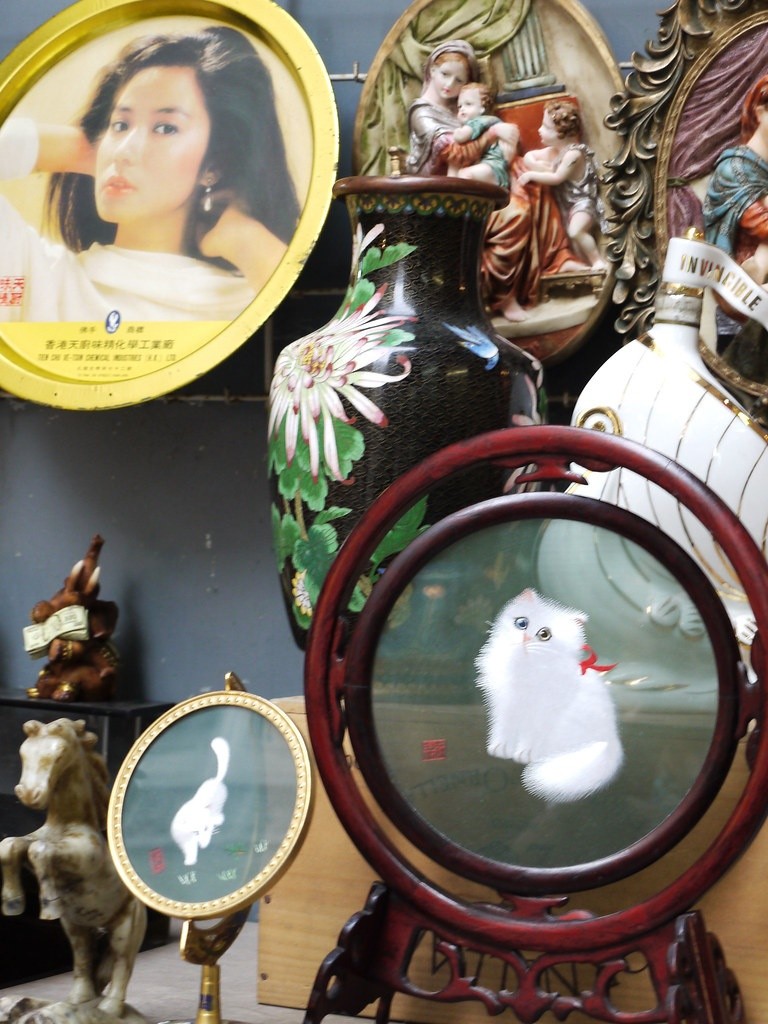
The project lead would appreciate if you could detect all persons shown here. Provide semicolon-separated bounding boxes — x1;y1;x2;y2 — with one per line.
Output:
445;82;517;186
404;39;591;322
518;98;606;272
0;27;300;321
700;73;768;430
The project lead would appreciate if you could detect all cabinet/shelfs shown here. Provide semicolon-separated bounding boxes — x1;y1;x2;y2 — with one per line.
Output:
0;686;177;991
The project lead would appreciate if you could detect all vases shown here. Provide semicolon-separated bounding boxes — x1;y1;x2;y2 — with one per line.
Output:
263;174;549;686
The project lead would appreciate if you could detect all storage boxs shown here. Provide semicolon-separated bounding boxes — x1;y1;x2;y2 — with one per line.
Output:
253;694;768;1024
